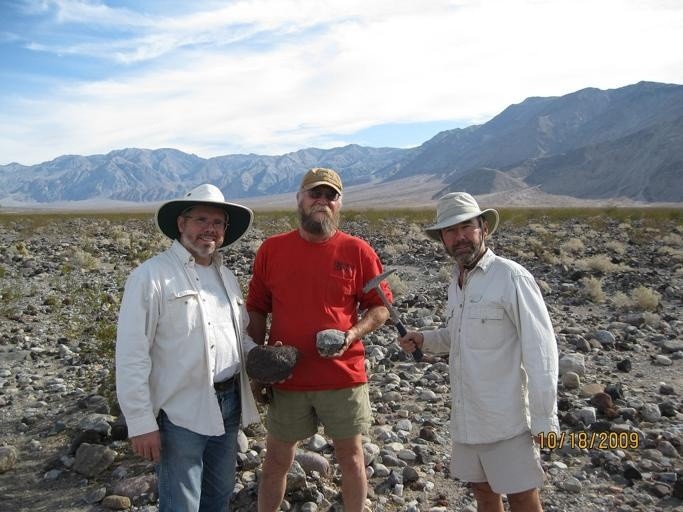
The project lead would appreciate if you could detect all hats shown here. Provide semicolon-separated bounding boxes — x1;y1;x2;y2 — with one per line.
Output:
421;190;501;243
295;167;344;199
153;182;254;252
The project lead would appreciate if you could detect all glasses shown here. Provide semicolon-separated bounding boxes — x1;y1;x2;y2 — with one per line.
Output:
304;189;342;203
180;213;229;229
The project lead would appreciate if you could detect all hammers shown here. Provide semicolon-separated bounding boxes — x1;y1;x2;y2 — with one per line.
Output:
361;268;423;361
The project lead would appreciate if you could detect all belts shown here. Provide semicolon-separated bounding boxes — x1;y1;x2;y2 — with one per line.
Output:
211;376;237;392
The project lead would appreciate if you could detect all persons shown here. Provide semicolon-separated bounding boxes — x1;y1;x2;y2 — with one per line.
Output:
397;192;562;511
243;167;392;511
114;184;295;511
